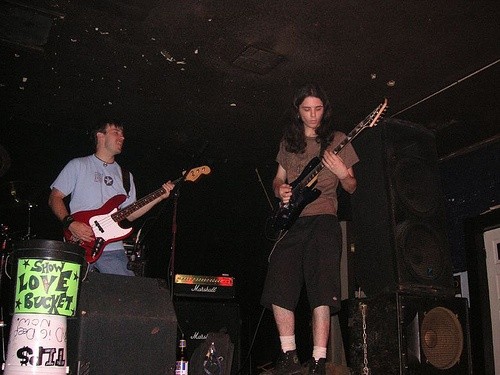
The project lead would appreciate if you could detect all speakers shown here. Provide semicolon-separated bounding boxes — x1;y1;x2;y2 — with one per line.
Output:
340;292;473;375
347;119;455;298
66;271;242;375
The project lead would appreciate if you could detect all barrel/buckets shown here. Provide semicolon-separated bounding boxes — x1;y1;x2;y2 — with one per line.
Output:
7;239;86;319
3;314;71;375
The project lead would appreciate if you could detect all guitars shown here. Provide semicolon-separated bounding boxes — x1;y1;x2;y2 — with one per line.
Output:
271;94;389;230
61;164;211;263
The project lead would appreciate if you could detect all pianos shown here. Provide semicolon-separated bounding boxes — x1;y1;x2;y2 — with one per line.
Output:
167;269;238;374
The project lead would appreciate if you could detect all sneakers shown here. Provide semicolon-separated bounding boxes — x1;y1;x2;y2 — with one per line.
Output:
259;349;304;375
308;356;327;375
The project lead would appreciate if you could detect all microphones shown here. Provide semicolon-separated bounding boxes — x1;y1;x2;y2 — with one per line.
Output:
130;229;142;261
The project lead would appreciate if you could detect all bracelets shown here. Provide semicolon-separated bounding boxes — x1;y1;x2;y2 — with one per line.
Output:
60;215;75;228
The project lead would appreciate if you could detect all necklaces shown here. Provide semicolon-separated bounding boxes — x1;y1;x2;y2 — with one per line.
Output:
95;153;116;168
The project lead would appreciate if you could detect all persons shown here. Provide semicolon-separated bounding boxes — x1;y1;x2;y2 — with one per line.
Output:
271;84;356;375
51;113;173;279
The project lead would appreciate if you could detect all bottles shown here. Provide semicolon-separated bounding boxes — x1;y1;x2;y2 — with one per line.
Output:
175;334;189;375
